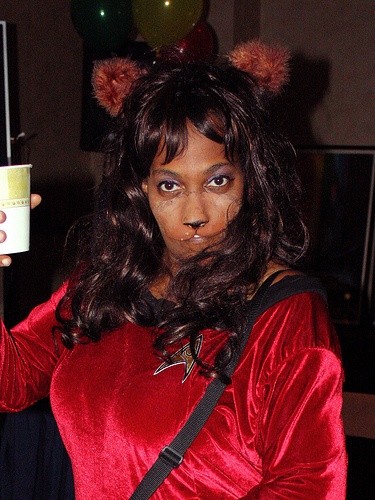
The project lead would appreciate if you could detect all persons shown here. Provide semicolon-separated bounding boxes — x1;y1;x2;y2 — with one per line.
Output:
1;49;351;500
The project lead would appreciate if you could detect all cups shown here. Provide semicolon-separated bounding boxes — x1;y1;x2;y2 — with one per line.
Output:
0;164;33;256
